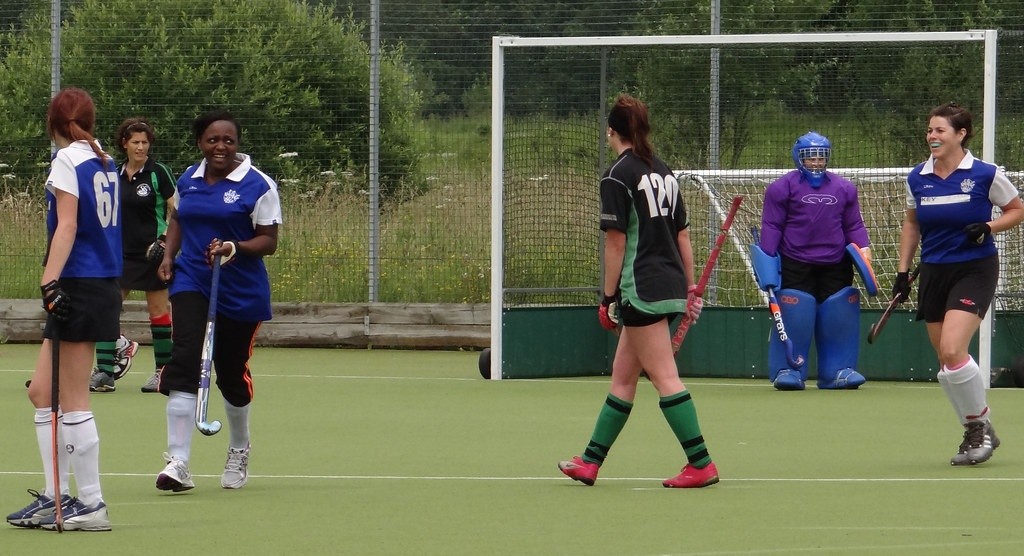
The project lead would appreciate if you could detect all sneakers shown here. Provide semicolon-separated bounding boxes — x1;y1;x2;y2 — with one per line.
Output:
558;457;600;486
155;458;195;492
662;461;720;488
142;368;161;392
114;333;139;380
951;419;1000;465
89;366;116;392
221;439;251;490
39;495;112;532
6;494;75;529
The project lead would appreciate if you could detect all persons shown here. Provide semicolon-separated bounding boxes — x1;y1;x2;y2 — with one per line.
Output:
89;111;283;491
890;102;1024;464
4;88;122;534
747;132;876;390
559;95;718;488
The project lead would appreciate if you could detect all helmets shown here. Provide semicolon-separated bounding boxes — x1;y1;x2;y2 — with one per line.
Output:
792;132;831;188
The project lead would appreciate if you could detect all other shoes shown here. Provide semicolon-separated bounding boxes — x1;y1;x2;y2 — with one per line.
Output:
818;368;866;390
773;369;805;390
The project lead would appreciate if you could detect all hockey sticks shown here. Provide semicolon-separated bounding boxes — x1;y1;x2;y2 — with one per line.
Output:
50;319;66;534
866;263;922;346
768;285;806;369
671;193;744;358
195;236;225;436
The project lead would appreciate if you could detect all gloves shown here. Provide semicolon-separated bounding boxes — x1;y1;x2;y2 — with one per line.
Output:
687;285;704;326
962;221;991;247
40;280;73;321
145;235;167;265
891;269;912;302
